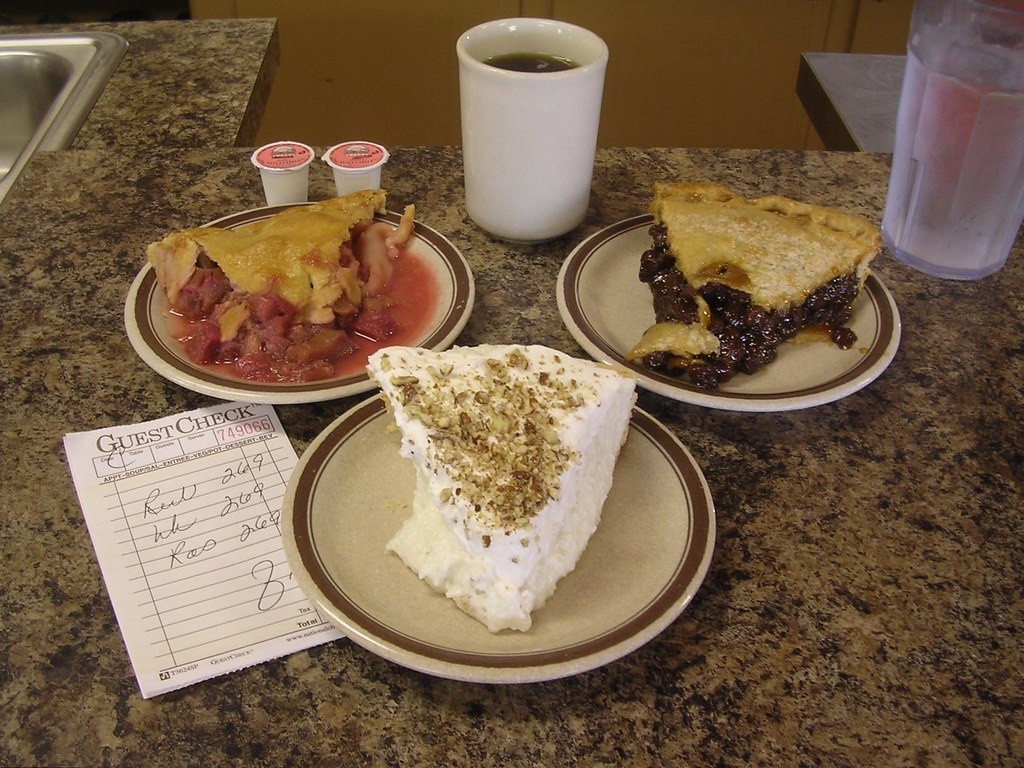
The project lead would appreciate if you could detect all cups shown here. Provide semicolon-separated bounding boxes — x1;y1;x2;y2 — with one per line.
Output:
880;0;1024;283
457;17;609;247
253;142;314;207
326;141;387;198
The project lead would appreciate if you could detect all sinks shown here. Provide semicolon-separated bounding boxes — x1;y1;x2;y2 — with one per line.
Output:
0;32;130;204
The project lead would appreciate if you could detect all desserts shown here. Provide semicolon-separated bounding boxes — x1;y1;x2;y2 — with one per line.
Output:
364;342;638;635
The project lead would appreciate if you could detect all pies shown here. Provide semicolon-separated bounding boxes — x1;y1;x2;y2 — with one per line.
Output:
625;180;884;393
143;188;414;382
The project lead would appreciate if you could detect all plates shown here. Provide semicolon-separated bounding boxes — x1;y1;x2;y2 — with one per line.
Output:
554;213;901;412
124;201;476;404
281;392;717;683
